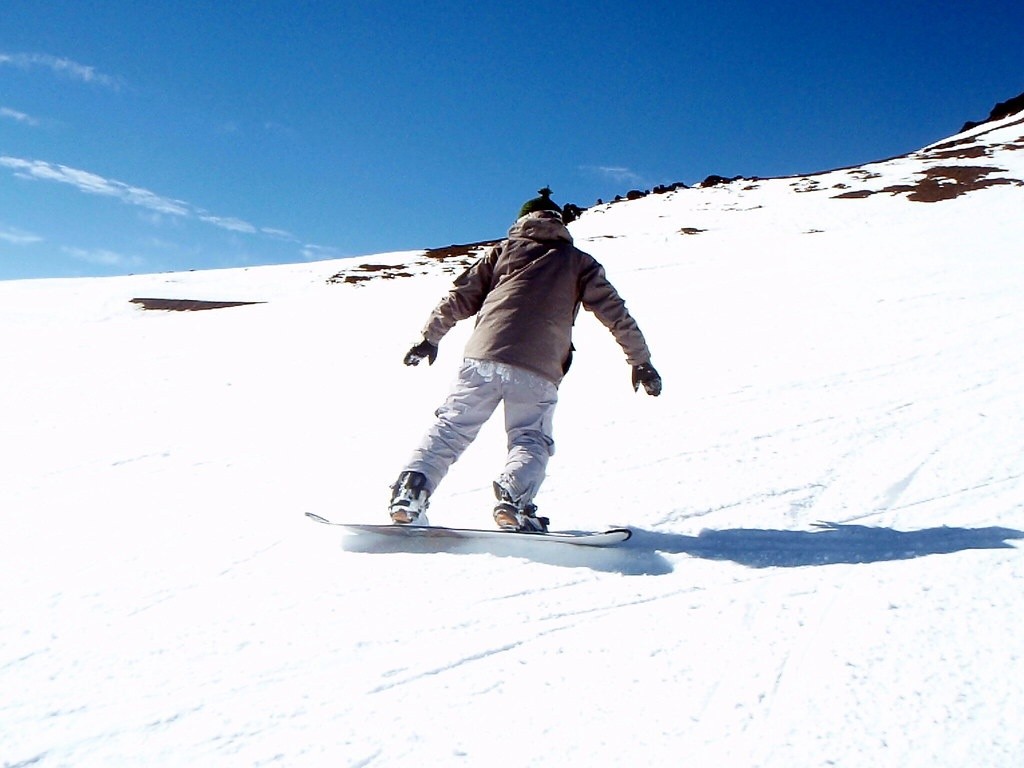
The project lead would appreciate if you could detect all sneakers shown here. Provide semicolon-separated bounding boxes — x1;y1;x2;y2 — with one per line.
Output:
390;470;430;529
493;491;549;532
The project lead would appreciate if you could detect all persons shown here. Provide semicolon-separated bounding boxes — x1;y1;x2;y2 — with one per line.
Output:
389;198;663;531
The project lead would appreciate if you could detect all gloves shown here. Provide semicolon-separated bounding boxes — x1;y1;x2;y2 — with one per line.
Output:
403;339;439;367
631;362;662;397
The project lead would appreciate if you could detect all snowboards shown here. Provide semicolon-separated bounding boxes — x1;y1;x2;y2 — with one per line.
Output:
305;510;633;547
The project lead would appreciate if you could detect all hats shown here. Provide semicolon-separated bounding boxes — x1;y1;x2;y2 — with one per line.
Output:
517;185;562;218
